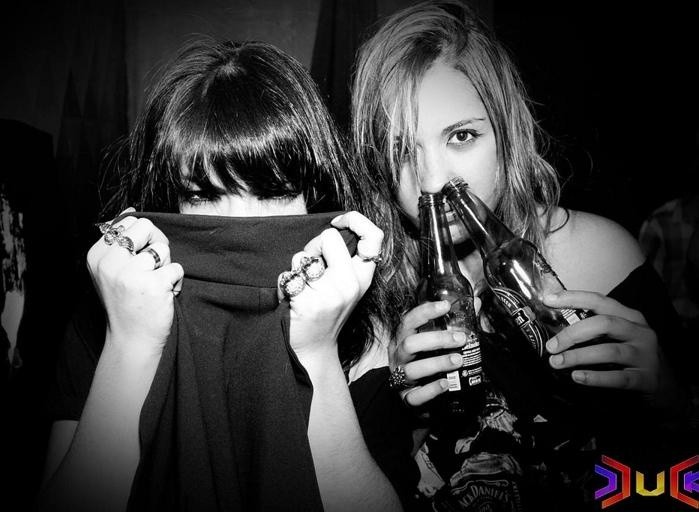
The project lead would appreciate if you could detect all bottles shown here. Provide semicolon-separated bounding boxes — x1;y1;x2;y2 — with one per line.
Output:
415;193;486;417
440;176;618;407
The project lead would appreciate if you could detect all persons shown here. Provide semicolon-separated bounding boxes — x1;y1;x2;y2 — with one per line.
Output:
638;197;699;326
351;2;697;511
40;35;421;511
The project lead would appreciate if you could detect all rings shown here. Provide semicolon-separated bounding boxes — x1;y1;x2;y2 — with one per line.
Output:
142;246;162;271
356;250;382;267
389;365;417;389
97;223;134;254
277;270;305;296
299;254;325;281
